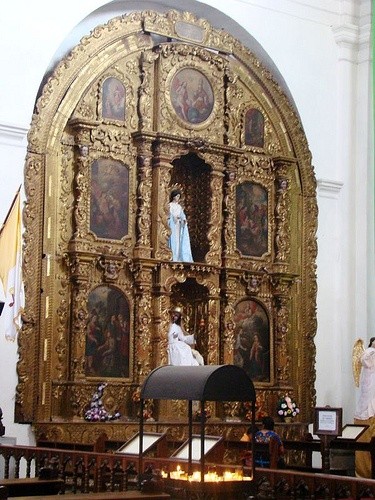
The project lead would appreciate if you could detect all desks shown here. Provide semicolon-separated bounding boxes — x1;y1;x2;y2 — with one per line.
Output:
35;421;305;446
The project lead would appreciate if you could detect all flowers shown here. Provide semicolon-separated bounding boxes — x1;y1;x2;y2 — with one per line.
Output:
83;383;121;422
277;393;299;417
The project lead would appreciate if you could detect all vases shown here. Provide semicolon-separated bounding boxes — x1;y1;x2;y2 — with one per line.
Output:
285;417;292;423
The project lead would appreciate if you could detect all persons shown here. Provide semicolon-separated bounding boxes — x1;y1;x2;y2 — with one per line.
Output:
241;416;286;484
166;190;194;263
167;308;205;369
352;337;375;421
